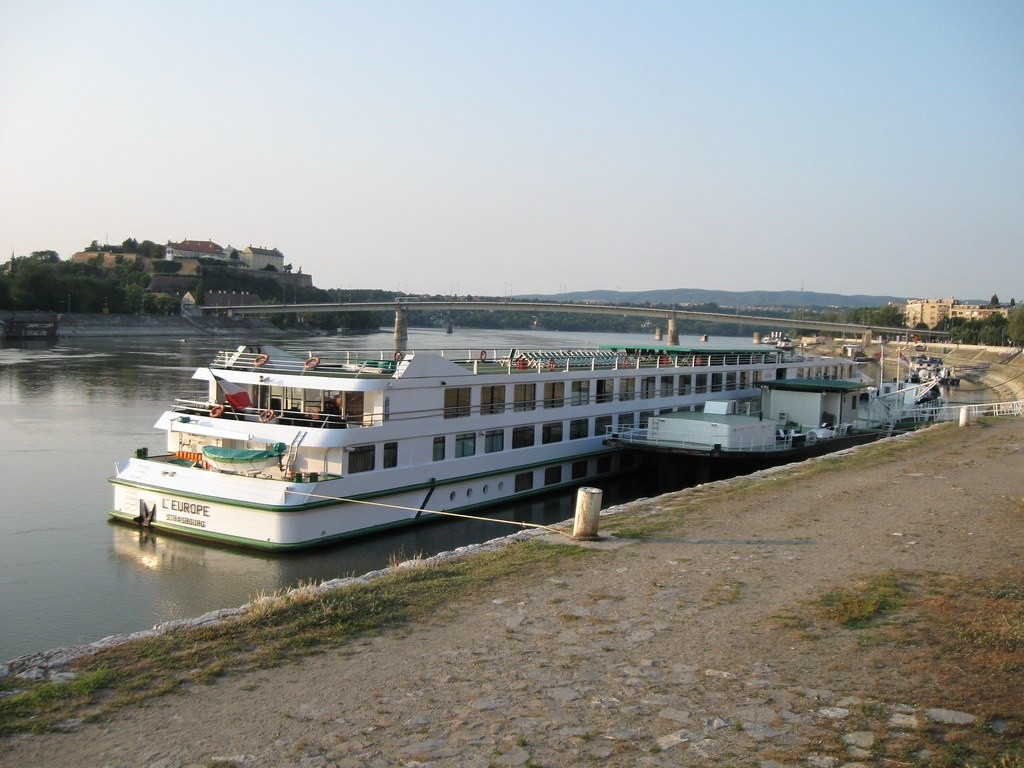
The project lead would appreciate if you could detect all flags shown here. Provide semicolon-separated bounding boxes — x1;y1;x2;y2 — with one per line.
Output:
900;353;912;368
875;352;881;361
215;375;252;410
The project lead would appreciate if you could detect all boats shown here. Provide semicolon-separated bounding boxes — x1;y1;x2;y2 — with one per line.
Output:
107;345;873;557
602;375;944;489
905;354;961;386
839;345;873;362
775;338;795;351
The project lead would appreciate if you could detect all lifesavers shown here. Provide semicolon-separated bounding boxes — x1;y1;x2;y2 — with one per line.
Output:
254;353;268;367
304;357;320;368
208;404;223;418
548;359;556;372
623;358;629;368
259;409;275;423
394;351;402;362
480;351;487;362
